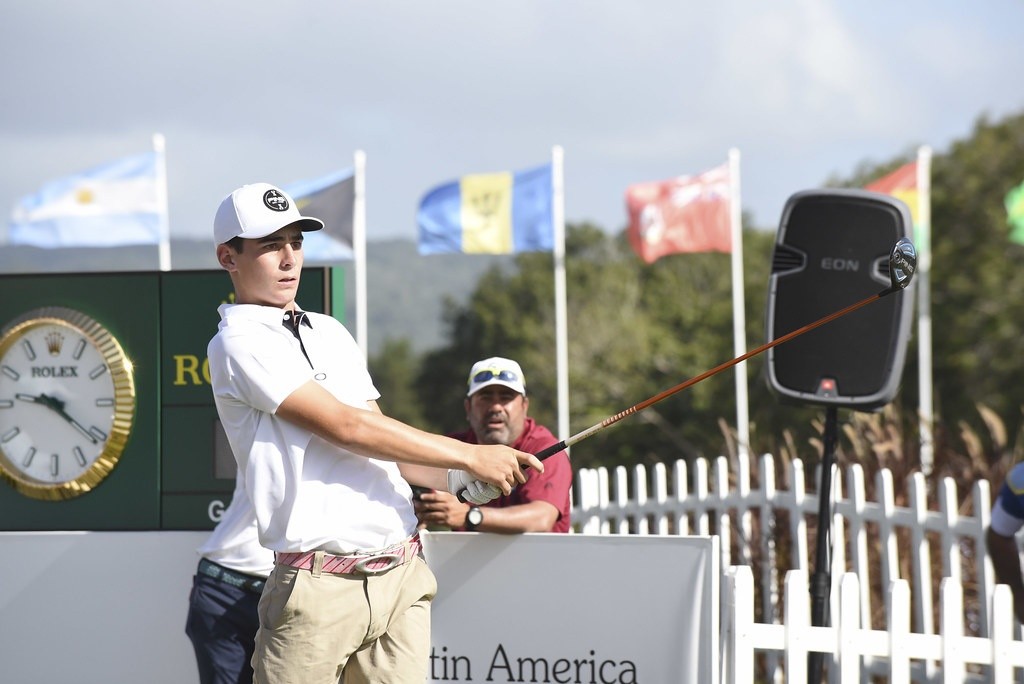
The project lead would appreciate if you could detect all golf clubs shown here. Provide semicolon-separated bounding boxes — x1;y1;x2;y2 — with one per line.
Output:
456;239;917;504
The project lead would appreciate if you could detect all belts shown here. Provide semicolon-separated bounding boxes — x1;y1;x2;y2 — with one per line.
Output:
274;530;424;574
197;559;266;595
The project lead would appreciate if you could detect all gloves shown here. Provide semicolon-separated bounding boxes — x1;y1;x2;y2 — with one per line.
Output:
446;468;503;506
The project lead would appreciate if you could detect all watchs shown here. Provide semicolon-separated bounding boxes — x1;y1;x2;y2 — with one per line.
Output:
462;502;485;531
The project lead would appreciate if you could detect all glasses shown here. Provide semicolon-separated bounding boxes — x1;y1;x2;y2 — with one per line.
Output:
467;367;526;386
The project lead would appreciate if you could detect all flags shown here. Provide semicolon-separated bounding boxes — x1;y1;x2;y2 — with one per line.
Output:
11;149;1024;272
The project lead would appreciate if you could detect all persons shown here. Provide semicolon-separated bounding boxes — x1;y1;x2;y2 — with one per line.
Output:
207;184;544;684
182;450;273;684
984;460;1024;623
406;357;571;531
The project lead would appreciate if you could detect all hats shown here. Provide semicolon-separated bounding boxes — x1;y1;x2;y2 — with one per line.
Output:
466;357;527;399
214;182;325;251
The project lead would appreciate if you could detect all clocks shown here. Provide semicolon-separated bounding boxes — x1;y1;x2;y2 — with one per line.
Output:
0;305;134;499
